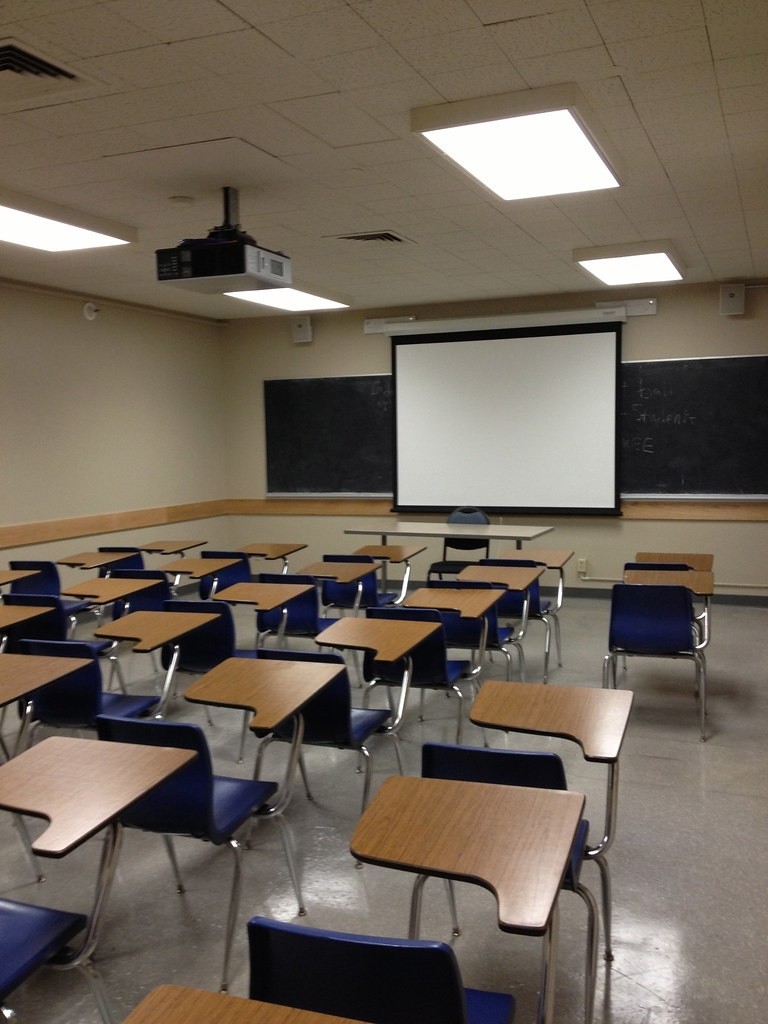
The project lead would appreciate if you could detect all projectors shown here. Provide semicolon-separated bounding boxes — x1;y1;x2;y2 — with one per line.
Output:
156;241;292;294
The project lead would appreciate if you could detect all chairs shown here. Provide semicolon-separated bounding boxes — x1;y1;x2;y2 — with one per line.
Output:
322;555;398;617
363;607;489;747
623;562;711;696
256;575;364;688
602;584;709;742
426;506;490;588
111;569;174;673
18;638;160;730
479;559;565;684
428;580;525;683
161;600;258;764
98;714;307;992
97;546;144;579
421;743;614;1024
199;551;252;606
0;898;115;1024
253;648;404;870
2;594;127;694
7;560;91;641
247;916;515;1024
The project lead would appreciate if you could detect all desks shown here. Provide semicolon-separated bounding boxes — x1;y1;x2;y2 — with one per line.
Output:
623;552;714;650
470;680;631;859
121;984;372;1024
0;652;94;709
212;583;314;649
314;617;441;736
57;552;164;605
403;587;506;681
0;736;197;971
0;570;55;628
350;775;586;1024
456;565;546;644
139;541;308;586
501;549;576;614
295;562;383;617
94;610;221;719
159;559;243;602
352;546;428;605
343;522;554;550
184;657;347;819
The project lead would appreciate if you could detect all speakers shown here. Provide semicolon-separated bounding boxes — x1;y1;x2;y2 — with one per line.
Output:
719;284;745;315
291;316;312;342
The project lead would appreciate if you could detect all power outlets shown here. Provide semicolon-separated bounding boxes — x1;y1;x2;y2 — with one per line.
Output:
578;559;588;573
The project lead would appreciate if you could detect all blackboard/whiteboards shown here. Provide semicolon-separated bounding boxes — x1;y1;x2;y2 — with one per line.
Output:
261;354;768;505
